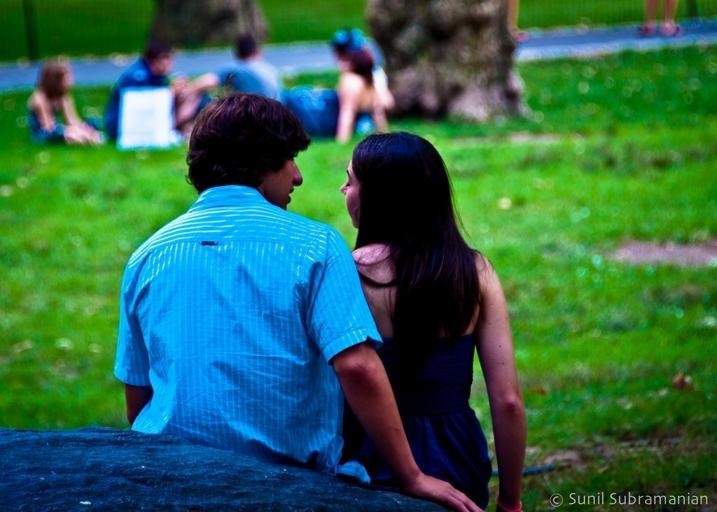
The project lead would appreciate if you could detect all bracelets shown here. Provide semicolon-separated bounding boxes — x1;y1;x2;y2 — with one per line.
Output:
494;493;523;512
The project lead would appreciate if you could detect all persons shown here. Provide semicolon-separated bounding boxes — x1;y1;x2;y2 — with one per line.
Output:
112;71;486;511
24;26;397;149
338;132;528;511
636;0;684;38
